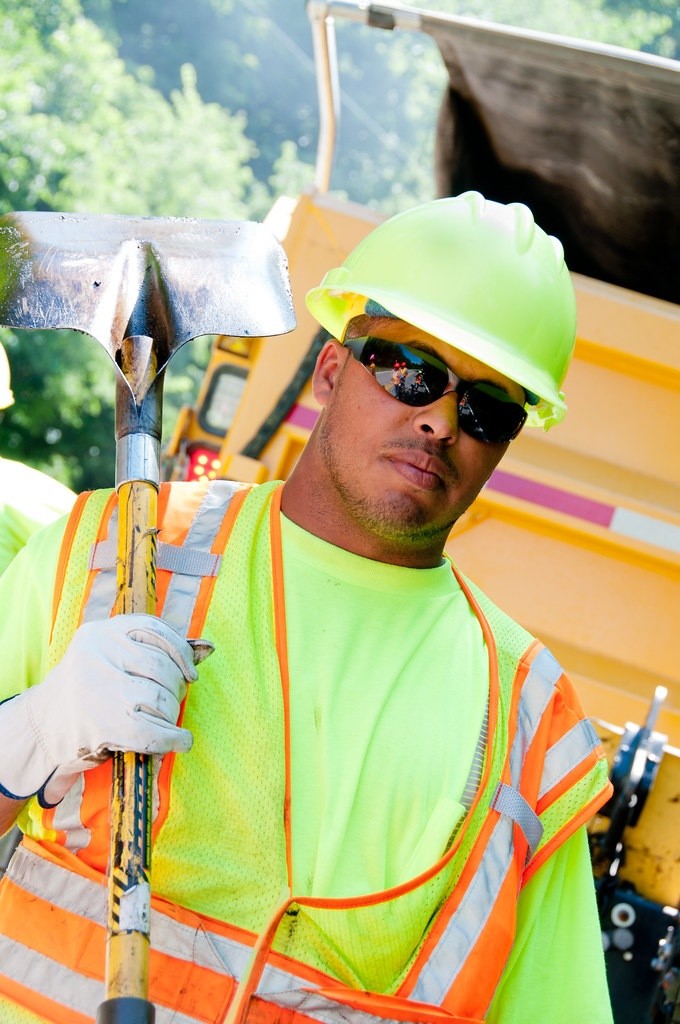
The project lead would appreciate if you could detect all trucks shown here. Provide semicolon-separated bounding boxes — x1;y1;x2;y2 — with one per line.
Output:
164;0;680;1024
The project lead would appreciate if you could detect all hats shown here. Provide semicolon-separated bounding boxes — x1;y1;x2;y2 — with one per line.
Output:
394;362;400;369
370;355;375;361
418;371;422;373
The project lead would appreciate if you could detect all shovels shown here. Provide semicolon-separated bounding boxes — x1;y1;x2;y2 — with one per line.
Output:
0;212;302;1024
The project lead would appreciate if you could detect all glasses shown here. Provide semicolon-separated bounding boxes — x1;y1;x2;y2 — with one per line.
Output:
343;336;528;445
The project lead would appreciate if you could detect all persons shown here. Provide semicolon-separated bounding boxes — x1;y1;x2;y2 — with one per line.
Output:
1;189;614;1024
1;345;81;571
368;351;424;401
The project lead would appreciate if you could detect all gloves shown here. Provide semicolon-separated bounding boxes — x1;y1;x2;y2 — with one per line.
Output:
0;613;215;809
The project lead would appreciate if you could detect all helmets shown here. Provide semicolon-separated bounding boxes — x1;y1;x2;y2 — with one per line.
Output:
0;342;15;410
305;191;578;432
401;362;406;367
